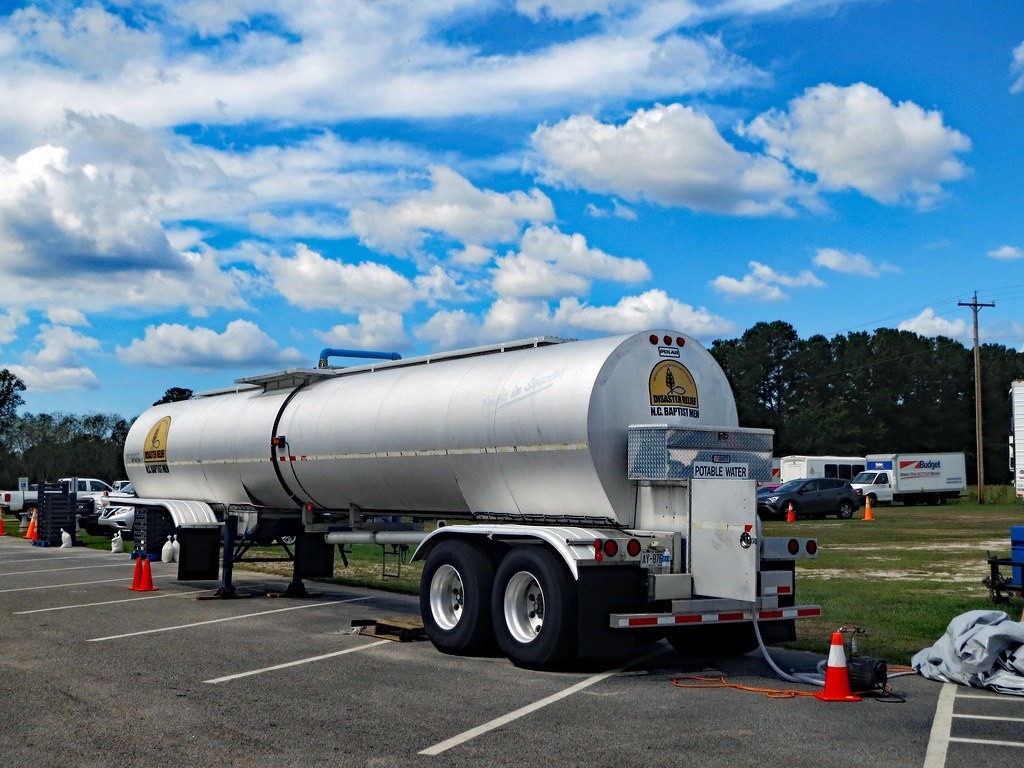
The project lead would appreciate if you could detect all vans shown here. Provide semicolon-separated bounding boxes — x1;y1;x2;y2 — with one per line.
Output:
98;481;135;541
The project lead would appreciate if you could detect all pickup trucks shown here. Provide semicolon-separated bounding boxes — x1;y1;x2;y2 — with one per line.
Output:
0;479;115;528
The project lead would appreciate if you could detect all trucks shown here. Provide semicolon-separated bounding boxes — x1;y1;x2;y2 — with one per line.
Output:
224;508;296;545
850;452;969;507
1008;380;1023;497
772;456;867;486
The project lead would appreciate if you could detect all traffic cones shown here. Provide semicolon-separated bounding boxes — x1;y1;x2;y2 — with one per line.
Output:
0;505;7;536
861;496;875;520
786;503;796;522
22;509;39;543
813;631;862;702
128;557;159;591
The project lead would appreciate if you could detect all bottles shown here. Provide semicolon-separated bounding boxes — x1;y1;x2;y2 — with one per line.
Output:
662;549;671;574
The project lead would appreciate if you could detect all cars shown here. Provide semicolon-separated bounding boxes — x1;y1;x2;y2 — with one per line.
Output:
757;477;860;519
